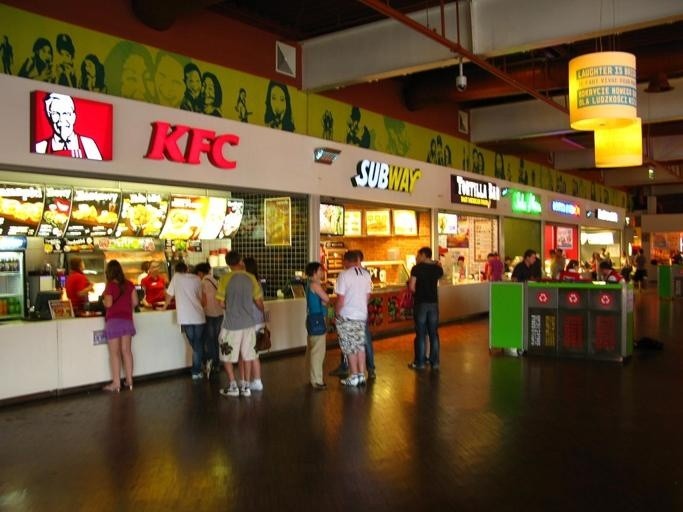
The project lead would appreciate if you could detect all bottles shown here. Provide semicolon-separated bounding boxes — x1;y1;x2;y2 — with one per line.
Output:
0;257;20;272
0;295;21;315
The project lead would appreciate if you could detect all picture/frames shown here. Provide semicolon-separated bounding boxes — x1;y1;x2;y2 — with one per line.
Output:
263;197;292;246
474;219;493;262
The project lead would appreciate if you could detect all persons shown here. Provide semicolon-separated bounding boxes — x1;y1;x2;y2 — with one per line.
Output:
158;249;377;397
449;246;682;285
407;246;443;371
64;255;94;317
137;261;148;285
141;260;168;306
100;260;137;395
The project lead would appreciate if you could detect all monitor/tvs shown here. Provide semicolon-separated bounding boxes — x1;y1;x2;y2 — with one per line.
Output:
35;290;62;311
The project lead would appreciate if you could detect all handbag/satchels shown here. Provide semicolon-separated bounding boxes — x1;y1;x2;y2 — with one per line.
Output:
202;315;223;343
256;326;271;350
306;313;326;335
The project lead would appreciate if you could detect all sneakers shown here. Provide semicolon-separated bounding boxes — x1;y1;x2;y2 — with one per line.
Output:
220;380;264;397
409;356;439;370
206;359;216;380
192;372;205;380
310;365;376;390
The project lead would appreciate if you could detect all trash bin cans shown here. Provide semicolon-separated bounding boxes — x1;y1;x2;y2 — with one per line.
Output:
673;276;683;296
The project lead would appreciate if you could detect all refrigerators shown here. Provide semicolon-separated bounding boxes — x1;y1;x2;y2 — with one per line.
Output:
0;235;28;322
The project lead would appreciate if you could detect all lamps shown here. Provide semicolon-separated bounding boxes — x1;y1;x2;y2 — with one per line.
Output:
569;1;637;131
594;0;643;168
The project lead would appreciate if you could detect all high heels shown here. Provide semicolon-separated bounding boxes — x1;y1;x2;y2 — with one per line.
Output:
124;382;133;391
102;386;121;394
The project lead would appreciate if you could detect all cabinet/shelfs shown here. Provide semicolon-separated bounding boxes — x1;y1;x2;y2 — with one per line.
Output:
0;251;23;319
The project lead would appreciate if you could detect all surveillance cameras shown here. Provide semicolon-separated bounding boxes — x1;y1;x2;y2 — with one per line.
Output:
455;76;467;92
